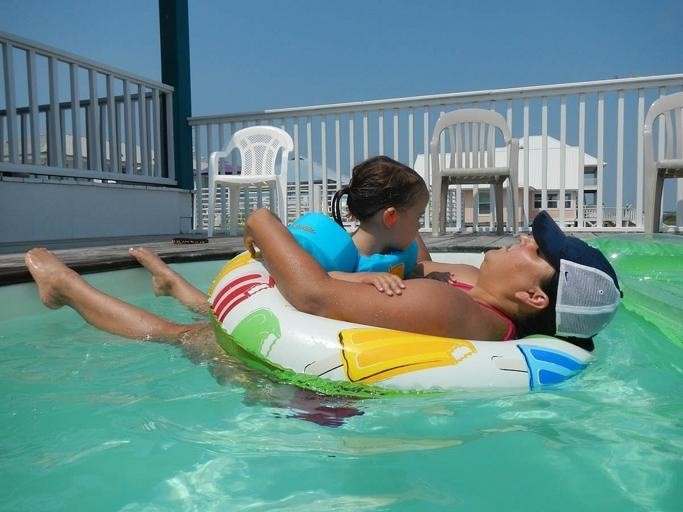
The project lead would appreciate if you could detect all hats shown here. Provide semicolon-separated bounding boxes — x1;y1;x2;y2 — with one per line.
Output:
532;210;623;340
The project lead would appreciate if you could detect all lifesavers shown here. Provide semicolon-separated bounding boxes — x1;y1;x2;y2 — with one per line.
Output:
207;245;592;399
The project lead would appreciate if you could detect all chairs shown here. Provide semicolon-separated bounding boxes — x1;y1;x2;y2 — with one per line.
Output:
642;91;683;237
430;108;520;237
207;125;294;238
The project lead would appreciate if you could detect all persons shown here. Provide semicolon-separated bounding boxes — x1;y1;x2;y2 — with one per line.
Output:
285;155;458;297
22;207;625;428
